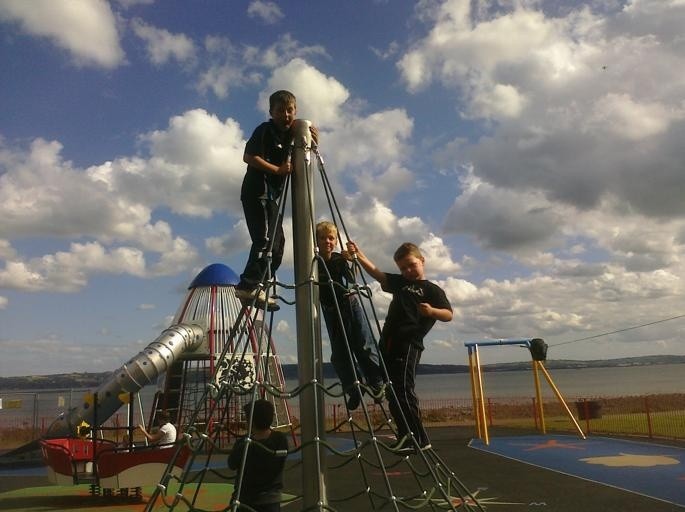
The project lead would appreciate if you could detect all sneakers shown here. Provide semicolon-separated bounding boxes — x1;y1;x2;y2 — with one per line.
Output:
242;297;280;312
399;440;431;453
235;286;275;304
372;382;386;403
390;429;415;452
347;384;366;410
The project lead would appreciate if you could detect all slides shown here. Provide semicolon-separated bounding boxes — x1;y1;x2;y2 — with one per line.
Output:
44;323;204;440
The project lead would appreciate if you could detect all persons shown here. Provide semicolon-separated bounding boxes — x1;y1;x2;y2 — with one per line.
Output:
138;409;178;450
316;220;387;409
235;89;319;312
226;397;288;512
345;239;453;454
